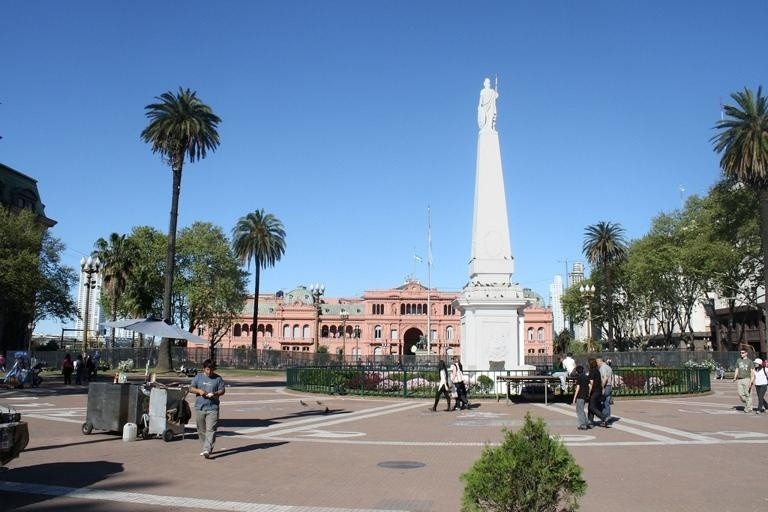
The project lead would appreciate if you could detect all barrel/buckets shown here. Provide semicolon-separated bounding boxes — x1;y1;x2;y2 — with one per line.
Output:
122;423;137;442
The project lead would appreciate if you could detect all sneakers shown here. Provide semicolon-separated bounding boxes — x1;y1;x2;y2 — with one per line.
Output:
200;451;209;459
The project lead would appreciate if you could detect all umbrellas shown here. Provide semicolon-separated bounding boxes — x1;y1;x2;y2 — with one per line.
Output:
96;317;208;382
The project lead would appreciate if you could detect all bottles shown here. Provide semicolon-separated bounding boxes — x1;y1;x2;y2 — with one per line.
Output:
112;372;119;384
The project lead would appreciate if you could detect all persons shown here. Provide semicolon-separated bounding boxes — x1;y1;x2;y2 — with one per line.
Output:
607;356;768;381
453;355;467;409
563;352;577;377
0;350;101;387
596;357;613;427
477;76;498;129
428;360;451;412
188;359;225;458
749;357;768;414
587;358;612;428
573;365;594;430
731;348;755;414
557;353;565;370
450;358;471;411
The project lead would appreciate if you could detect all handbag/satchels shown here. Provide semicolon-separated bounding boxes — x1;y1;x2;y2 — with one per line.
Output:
451;371;464;383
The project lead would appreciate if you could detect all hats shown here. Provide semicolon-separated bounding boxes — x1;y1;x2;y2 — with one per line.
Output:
753;359;762;365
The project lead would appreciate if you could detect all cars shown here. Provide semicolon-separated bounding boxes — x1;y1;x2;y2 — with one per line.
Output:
278;362;298;369
0;403;30;465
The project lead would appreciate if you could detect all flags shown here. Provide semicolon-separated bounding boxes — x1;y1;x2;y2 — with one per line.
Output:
427;209;433;269
414;254;424;263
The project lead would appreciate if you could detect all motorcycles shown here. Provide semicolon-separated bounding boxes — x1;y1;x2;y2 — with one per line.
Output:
3;352;43;388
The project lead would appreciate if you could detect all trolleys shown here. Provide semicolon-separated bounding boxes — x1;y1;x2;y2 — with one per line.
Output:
142;386;189;442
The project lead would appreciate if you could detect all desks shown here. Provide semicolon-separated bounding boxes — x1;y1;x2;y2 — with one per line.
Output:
499;375;583;407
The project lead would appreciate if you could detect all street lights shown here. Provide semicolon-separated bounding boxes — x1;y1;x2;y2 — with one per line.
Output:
80;256;100;362
579;283;596;353
353;324;361;361
310;282;325;353
339;310;349;363
28;322;35;354
